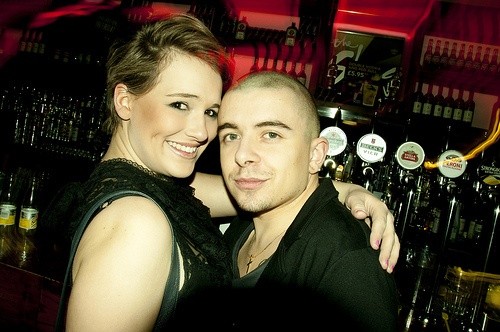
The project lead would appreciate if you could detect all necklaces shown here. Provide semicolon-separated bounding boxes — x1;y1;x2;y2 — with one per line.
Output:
246;230;285;273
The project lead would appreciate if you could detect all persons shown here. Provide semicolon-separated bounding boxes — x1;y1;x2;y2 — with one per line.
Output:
48;14;399;332
222;70;400;332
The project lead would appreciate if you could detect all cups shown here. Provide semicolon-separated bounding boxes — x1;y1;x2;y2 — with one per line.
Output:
472;297;500;332
442;265;476;316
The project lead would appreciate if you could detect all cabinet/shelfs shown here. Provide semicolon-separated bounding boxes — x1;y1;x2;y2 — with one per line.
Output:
398;30;500;146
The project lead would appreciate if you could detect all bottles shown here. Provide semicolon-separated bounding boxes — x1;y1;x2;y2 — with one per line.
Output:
411;83;476;130
249;57;307;87
423;39;500;76
0;171;49;237
387;65;401;102
319;141;500;244
326;55;338;90
0;86;109;145
285;21;298;47
235;16;247;41
